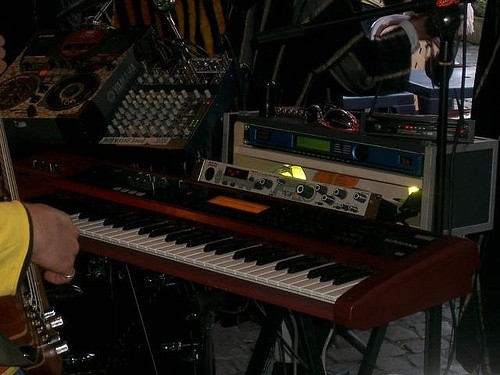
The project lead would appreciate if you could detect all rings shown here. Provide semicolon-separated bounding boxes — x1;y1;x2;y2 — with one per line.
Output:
63;270;75;279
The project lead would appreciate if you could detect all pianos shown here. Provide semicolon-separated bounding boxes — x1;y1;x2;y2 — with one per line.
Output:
15;152;483;329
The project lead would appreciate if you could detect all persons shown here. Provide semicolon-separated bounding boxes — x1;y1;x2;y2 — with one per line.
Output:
222;0;436;119
0;200;81;375
